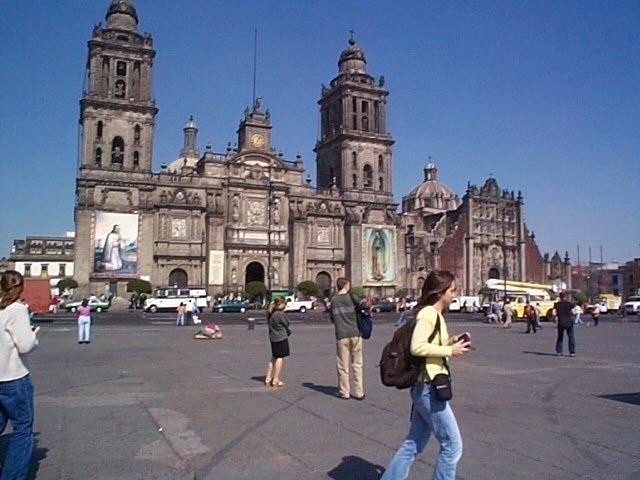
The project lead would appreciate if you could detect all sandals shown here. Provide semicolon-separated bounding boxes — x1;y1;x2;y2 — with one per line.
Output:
271;378;285;388
264;377;273;386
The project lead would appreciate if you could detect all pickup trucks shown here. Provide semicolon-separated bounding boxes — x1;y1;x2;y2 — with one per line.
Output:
284;294;314;314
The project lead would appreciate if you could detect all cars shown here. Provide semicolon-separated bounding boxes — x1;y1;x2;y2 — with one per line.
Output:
63;295;111;314
211;297;252;313
370;293;640;320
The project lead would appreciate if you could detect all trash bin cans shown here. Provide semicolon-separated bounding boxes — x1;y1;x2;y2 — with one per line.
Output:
248;317;255;330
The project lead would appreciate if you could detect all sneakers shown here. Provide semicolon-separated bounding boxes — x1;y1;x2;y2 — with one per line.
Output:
334;392;350;400
570;353;576;358
349;393;365;401
556;352;562;356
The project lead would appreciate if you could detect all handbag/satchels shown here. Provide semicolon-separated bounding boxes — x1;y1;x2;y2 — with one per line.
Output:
430;373;454;402
347;291;373;340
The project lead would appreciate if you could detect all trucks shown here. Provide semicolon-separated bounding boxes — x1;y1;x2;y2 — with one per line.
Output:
141;287;210;314
20;277;61;318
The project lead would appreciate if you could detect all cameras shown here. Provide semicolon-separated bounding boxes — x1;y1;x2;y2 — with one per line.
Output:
458;333;470;349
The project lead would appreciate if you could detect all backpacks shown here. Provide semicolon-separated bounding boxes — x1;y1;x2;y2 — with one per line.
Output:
377;302;440;391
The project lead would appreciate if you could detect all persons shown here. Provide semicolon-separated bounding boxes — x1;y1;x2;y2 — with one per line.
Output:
245;162;268;180
263;295;293;388
395;296;408;326
47;291;150;315
75;298;92;345
378;270;471;480
371;230;387;279
94;223;128;272
553;291;577;357
266;294;287;322
462;299;600;335
176;291;242;340
328;277;372;401
0;269;42;479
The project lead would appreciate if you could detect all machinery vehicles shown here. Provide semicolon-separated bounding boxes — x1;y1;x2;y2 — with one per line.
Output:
480;279;565;318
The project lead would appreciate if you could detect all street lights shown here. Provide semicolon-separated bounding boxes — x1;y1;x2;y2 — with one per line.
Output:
265;179;280;293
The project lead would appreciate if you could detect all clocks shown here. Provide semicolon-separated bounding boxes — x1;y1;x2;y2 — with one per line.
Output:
250;133;265;148
